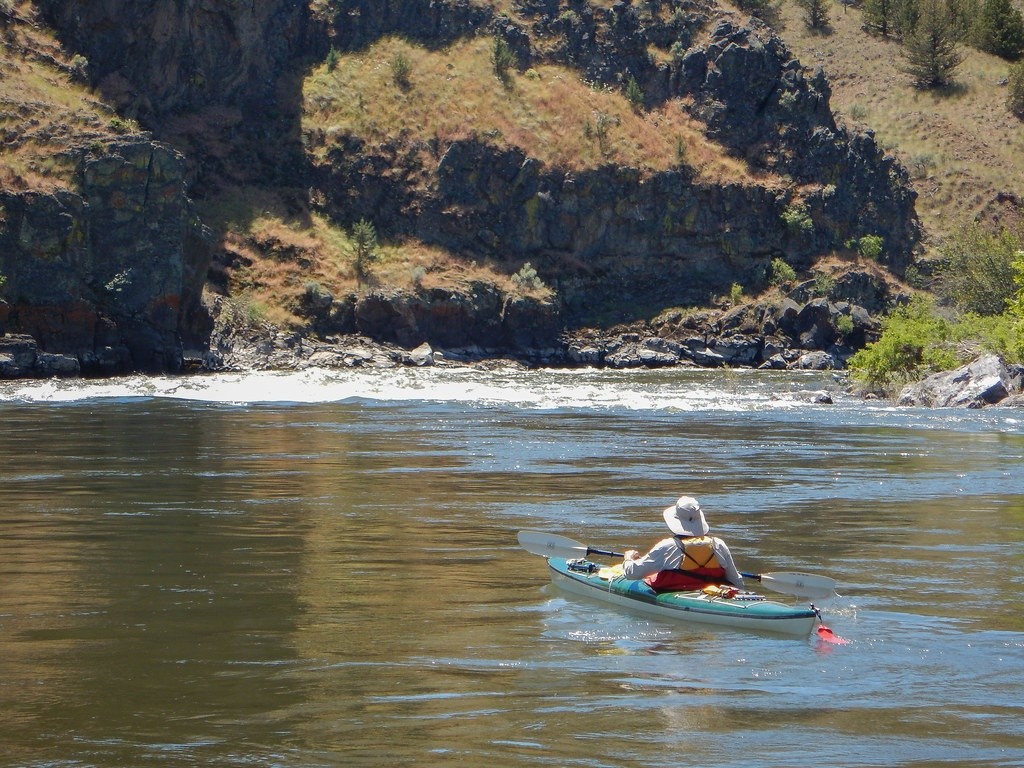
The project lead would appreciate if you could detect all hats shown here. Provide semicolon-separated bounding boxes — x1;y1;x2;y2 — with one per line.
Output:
663;495;709;536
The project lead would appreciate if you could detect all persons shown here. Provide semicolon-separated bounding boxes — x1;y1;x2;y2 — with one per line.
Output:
623;496;746;591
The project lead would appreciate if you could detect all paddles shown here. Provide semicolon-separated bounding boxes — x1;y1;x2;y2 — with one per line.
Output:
516;530;838;598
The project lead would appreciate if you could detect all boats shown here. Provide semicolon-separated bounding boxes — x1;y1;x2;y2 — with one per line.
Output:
545;556;817;638
